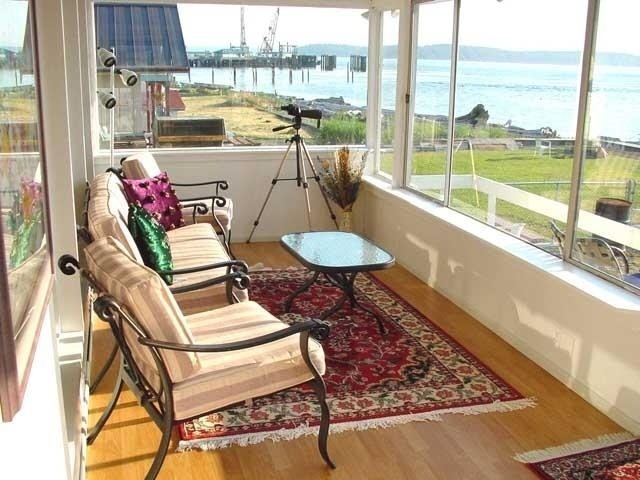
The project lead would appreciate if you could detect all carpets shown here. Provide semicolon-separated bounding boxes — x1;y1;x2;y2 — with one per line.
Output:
173;260;537;454
508;430;640;480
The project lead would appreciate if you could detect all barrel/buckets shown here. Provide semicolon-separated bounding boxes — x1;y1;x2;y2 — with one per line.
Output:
591;198;632;257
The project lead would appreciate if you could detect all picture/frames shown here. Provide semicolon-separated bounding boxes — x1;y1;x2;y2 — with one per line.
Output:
2;0;58;423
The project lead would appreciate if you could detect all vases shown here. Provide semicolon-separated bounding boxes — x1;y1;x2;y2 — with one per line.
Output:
339;211;356;232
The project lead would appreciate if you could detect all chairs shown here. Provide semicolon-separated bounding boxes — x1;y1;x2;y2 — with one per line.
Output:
547;221;640;289
74;171;251;394
118;151;239;261
59;236;336;480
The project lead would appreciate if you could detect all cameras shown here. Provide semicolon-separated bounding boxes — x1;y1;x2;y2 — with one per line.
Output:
272;103;323;133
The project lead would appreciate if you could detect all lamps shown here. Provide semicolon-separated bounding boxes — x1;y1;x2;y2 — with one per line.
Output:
95;44;140;169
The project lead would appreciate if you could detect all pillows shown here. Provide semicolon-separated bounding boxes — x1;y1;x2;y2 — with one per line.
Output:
122;171;184;286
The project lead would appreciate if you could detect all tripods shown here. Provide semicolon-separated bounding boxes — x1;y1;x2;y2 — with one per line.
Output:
246;133;340;244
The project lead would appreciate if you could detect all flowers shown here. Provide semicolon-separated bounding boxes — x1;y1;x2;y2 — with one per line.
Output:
315;145;373;210
